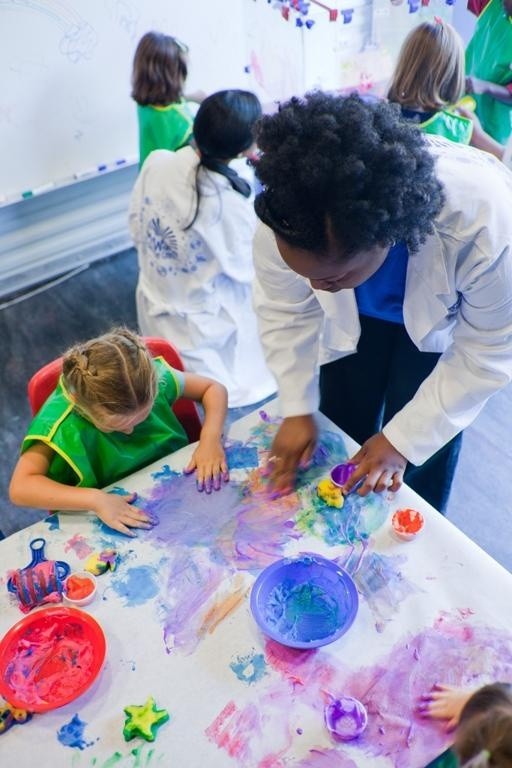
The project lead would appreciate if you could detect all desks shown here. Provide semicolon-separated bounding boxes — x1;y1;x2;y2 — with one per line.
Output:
1;392;512;762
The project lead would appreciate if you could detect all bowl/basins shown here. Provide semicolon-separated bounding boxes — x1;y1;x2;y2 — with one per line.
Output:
248;553;360;651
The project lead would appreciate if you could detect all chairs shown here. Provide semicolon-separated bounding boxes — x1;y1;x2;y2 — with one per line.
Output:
26;339;205;516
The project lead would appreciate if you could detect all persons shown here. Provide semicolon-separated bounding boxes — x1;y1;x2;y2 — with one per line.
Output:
456;100;511;170
128;29;203;176
128;89;278;410
7;320;231;540
465;0;512;143
385;13;468;126
417;678;511;768
244;88;510;517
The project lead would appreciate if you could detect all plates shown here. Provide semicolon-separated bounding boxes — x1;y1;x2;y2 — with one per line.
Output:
2;605;108;715
